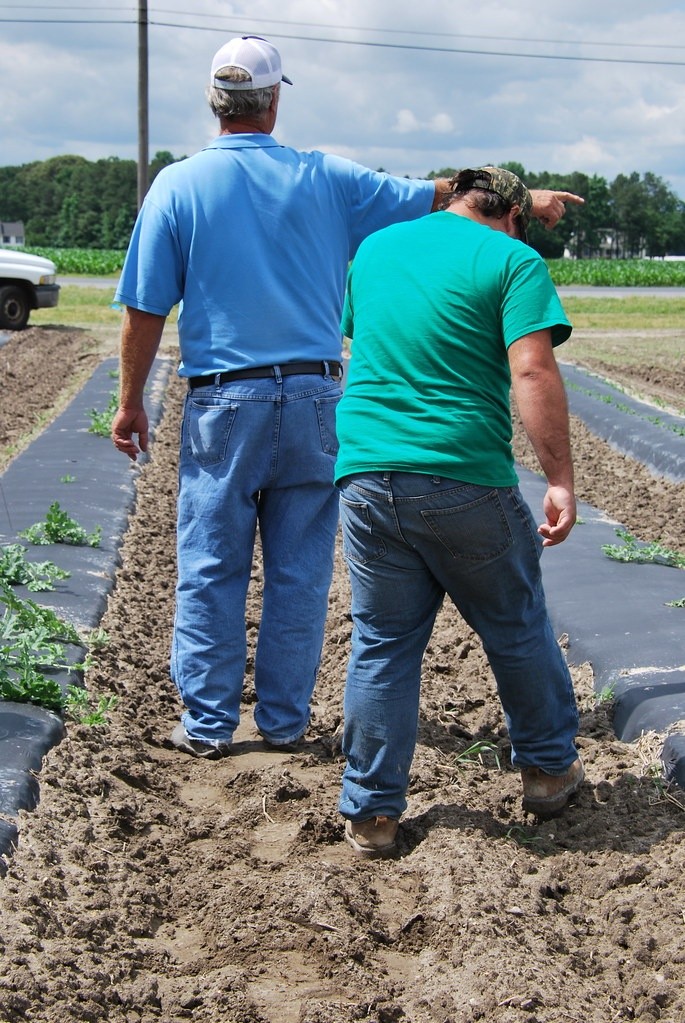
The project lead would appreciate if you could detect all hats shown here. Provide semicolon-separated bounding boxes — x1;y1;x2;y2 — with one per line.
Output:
211;35;294;90
448;167;532;245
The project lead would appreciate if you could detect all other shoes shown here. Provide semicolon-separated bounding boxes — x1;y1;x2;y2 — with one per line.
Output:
252;704;305;751
345;815;399;861
169;723;229;759
521;755;584;815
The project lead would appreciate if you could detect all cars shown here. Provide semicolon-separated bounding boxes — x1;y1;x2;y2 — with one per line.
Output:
0;248;61;332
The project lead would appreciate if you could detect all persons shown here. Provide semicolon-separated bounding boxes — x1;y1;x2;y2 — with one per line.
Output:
109;36;585;862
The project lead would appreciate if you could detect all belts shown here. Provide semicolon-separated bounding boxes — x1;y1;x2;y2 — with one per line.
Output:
189;360;340;390
